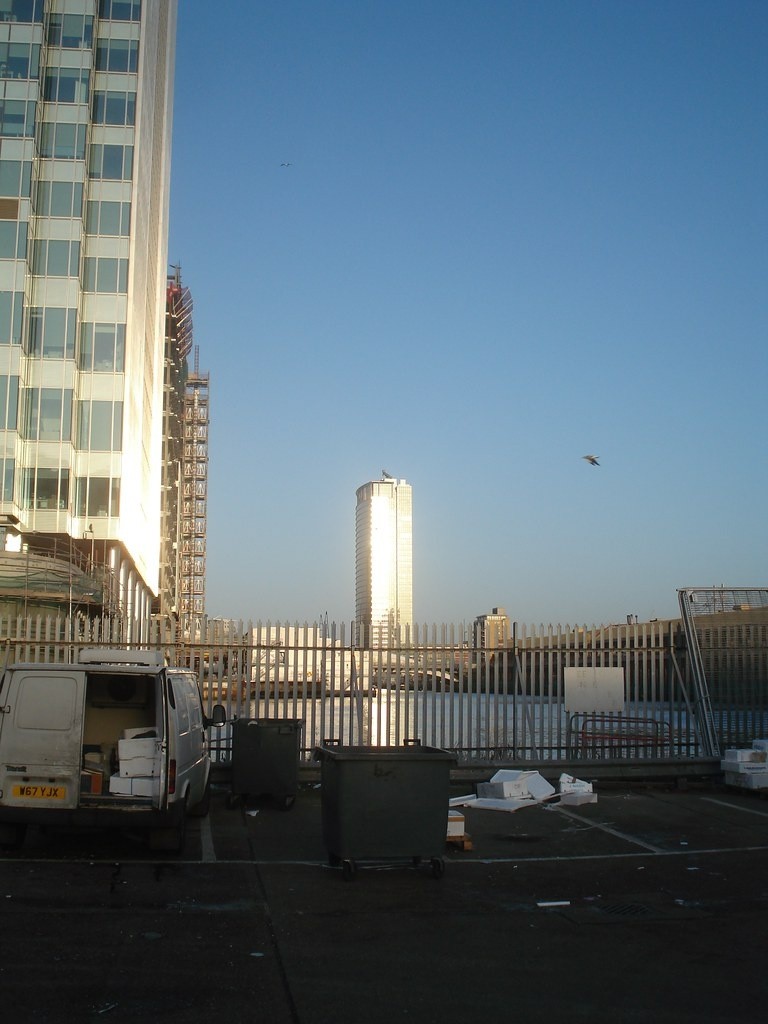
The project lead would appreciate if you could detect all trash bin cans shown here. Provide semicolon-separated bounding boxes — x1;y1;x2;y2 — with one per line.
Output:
313;739;461;877
231;717;306;810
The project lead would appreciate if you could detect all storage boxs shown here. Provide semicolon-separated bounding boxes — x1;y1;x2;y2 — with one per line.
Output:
720;739;768;790
78;728;161;798
477;768;597;806
447;811;465;836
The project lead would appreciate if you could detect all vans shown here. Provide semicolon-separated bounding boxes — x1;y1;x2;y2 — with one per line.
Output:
0;649;227;841
